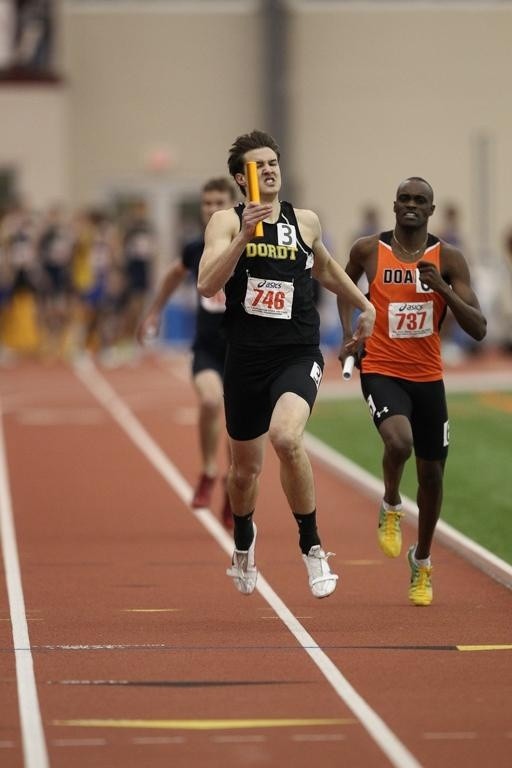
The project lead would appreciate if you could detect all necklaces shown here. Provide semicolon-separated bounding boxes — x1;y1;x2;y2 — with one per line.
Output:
392;229;429;255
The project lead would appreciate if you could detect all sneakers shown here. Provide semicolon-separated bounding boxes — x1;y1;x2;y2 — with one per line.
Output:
406;546;434;606
301;543;337;598
376;499;403;558
191;473;233;530
230;521;258;594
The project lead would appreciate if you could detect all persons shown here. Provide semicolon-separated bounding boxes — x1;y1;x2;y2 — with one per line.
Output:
434;198;466;250
0;192;158;373
357;198;386;240
335;177;488;608
196;131;376;599
134;177;240;528
